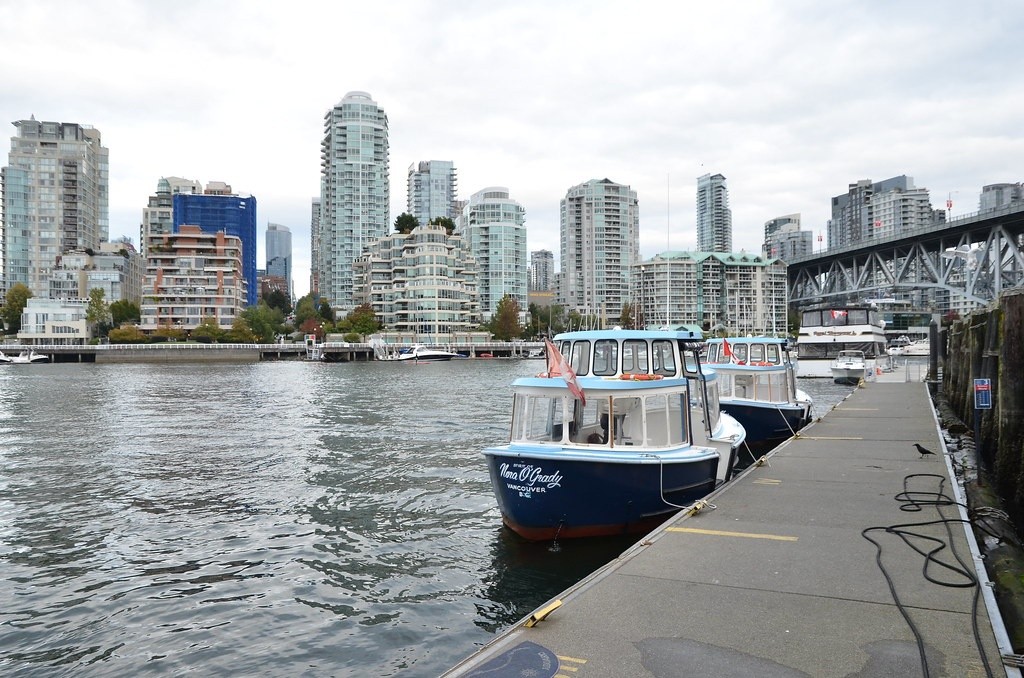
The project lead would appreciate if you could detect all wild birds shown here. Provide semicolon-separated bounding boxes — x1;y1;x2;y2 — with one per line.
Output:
913;443;937;459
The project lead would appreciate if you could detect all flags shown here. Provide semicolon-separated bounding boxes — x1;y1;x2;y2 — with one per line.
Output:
544;339;586;408
831;310;847;318
723;339;744;365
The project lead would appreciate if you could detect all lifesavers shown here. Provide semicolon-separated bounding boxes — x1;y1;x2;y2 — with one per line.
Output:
751;361;774;366
700;362;716;364
619;373;664;380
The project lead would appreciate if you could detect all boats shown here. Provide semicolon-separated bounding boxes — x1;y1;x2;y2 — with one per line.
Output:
0;347;49;364
388;343;458;361
700;337;813;448
796;302;895;378
831;349;876;384
885;334;930;356
481;324;746;540
